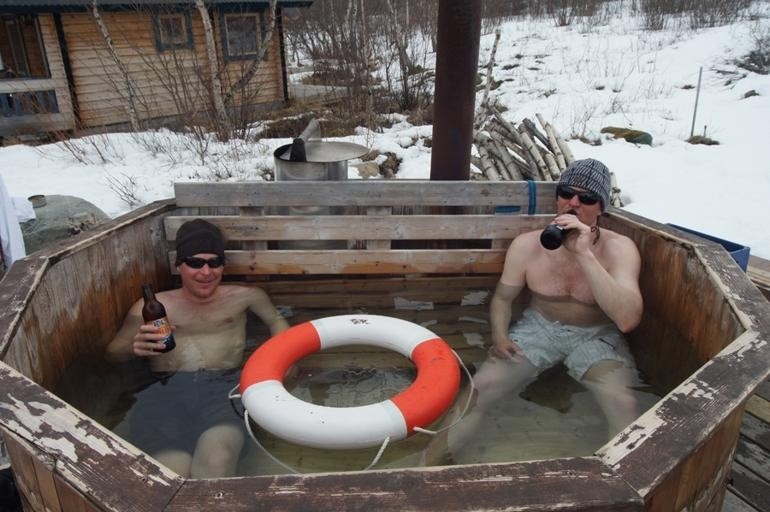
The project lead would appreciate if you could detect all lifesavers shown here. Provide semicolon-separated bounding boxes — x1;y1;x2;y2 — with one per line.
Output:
239;314;461;449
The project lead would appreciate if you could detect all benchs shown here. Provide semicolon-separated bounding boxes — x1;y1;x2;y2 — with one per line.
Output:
164;181;572;380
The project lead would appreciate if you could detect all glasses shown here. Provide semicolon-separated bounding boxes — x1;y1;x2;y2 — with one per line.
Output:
556;186;600;206
181;255;225;269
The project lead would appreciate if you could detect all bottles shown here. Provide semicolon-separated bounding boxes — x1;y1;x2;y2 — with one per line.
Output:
538;212;578;250
140;280;176;354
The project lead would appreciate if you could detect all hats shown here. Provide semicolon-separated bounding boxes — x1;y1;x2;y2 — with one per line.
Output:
558;158;612;213
175;218;225;266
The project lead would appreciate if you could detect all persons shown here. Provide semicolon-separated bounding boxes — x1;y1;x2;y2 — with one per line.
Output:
103;219;292;479
419;158;644;467
